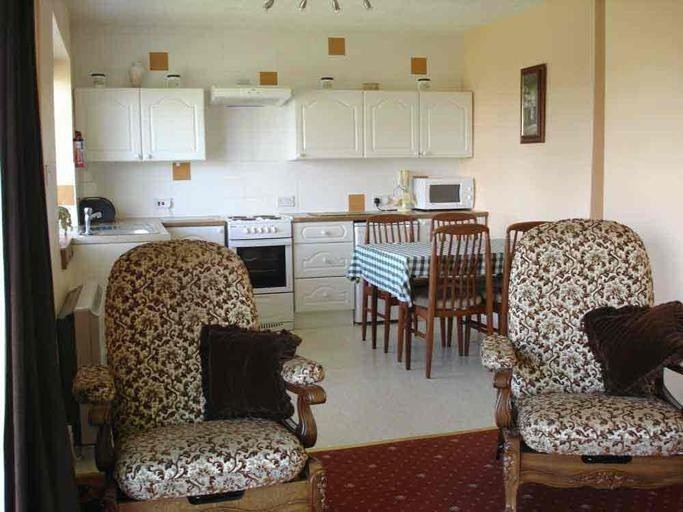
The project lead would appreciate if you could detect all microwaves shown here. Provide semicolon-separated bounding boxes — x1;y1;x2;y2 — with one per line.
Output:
413;176;477;209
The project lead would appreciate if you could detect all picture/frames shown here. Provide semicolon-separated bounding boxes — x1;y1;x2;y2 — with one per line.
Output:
518;63;547;145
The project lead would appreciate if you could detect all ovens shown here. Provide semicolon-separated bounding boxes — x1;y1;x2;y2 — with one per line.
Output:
228;221;296;293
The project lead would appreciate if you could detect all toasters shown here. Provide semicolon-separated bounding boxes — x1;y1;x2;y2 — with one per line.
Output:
78;198;115;225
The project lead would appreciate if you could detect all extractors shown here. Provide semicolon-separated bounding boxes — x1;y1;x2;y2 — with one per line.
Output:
209;86;293;107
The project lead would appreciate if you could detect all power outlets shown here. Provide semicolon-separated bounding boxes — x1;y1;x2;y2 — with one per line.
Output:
155;198;171;210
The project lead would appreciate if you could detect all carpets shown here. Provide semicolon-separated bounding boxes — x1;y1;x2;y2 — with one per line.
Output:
70;426;683;510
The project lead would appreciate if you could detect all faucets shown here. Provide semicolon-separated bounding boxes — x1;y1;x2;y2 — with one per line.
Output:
82;207;102;234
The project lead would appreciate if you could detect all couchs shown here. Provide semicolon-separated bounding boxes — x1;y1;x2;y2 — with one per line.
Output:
72;240;330;511
480;218;683;508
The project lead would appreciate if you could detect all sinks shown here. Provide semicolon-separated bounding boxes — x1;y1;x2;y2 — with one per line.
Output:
89;224;160;235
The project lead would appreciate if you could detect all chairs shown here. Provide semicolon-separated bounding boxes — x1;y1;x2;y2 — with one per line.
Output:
466;221;553;359
399;224;493;378
362;212;421;340
430;212;481;347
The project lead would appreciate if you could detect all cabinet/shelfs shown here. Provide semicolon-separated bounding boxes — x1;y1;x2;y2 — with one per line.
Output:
286;88;475;159
357;221;488;327
294;218;356;313
165;226;225;250
74;86;207;163
254;294;295;331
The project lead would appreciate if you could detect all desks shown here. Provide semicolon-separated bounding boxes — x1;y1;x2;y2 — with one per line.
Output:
347;238;525;362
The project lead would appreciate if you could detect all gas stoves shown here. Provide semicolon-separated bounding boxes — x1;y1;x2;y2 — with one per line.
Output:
226;213;292;220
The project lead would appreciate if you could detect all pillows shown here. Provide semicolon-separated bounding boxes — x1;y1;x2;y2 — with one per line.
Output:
199;323;303;421
585;299;683;393
